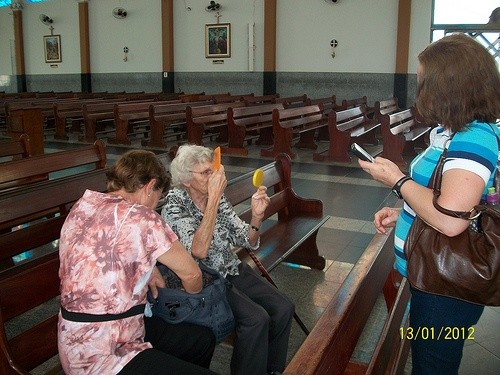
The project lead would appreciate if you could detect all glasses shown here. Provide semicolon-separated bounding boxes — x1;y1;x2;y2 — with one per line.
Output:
185;167;212;176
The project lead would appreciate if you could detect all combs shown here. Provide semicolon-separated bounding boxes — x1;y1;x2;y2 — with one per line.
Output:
215;146;221;171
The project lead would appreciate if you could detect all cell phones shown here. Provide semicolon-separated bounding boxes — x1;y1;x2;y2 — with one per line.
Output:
351;143;374;163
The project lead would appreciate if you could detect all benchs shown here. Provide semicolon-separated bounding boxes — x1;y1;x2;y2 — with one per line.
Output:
0;91;438;375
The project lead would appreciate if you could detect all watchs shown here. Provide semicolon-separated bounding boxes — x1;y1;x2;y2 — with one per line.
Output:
392;176;413;200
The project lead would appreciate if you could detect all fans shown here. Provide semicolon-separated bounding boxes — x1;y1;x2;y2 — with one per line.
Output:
111;6;128;19
39;14;53;26
205;1;220;12
205;23;231;58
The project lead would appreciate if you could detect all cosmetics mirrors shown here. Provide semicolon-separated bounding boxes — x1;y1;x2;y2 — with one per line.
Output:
252;169;264;188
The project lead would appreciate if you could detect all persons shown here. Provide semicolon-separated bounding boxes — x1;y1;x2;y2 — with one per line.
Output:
57;150;218;375
357;34;498;375
158;143;295;375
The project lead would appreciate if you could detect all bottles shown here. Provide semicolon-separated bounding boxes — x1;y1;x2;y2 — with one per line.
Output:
486;187;500;212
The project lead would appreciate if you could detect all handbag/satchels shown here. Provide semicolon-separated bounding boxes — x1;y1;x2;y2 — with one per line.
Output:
147;260;235;346
404;122;500;307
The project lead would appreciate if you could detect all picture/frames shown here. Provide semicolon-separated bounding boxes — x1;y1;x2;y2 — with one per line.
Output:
43;34;62;63
